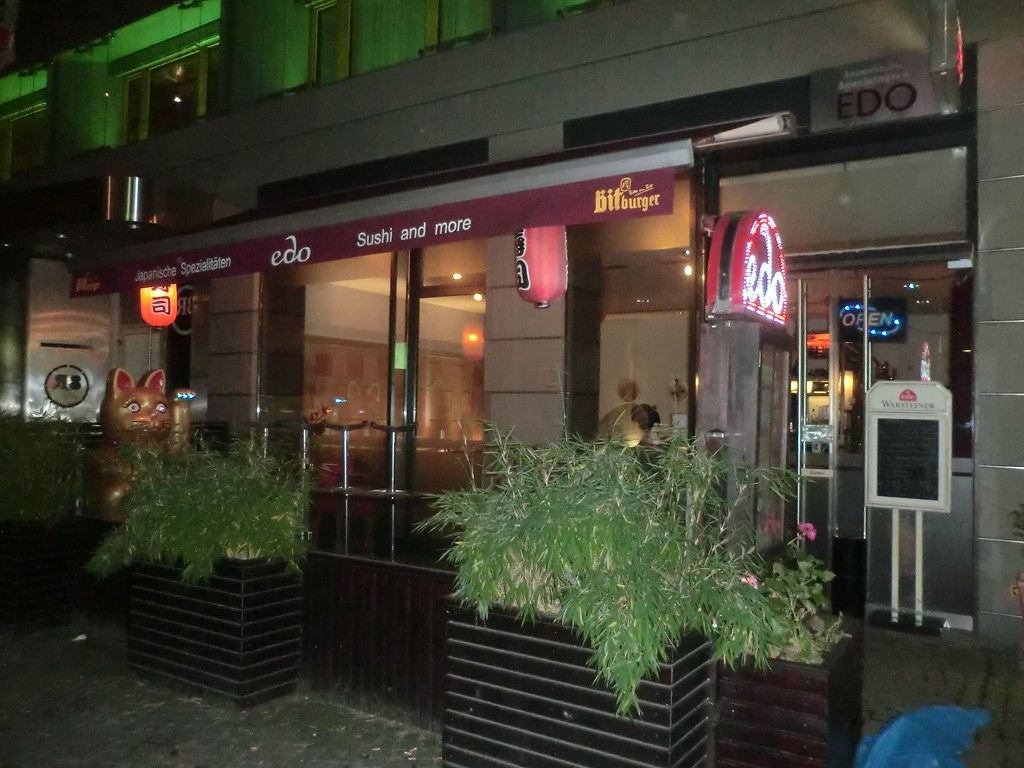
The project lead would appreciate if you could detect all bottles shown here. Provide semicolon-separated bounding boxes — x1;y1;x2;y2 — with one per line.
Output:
788;422;795;450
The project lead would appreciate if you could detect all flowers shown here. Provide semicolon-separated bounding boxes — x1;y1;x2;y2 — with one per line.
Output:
716;521;852;665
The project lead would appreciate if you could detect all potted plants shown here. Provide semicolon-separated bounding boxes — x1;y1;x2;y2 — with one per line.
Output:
409;372;818;768
86;412;318;715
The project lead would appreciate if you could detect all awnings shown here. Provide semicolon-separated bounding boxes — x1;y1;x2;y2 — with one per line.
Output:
67;135;695;300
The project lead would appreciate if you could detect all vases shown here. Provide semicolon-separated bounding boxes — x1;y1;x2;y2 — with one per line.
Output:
713;617;862;768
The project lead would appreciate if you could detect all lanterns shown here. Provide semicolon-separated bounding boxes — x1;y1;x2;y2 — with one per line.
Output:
140;284;177;331
515;224;569;308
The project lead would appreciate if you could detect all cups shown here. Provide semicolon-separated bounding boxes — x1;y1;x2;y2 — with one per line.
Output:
811;442;821;453
